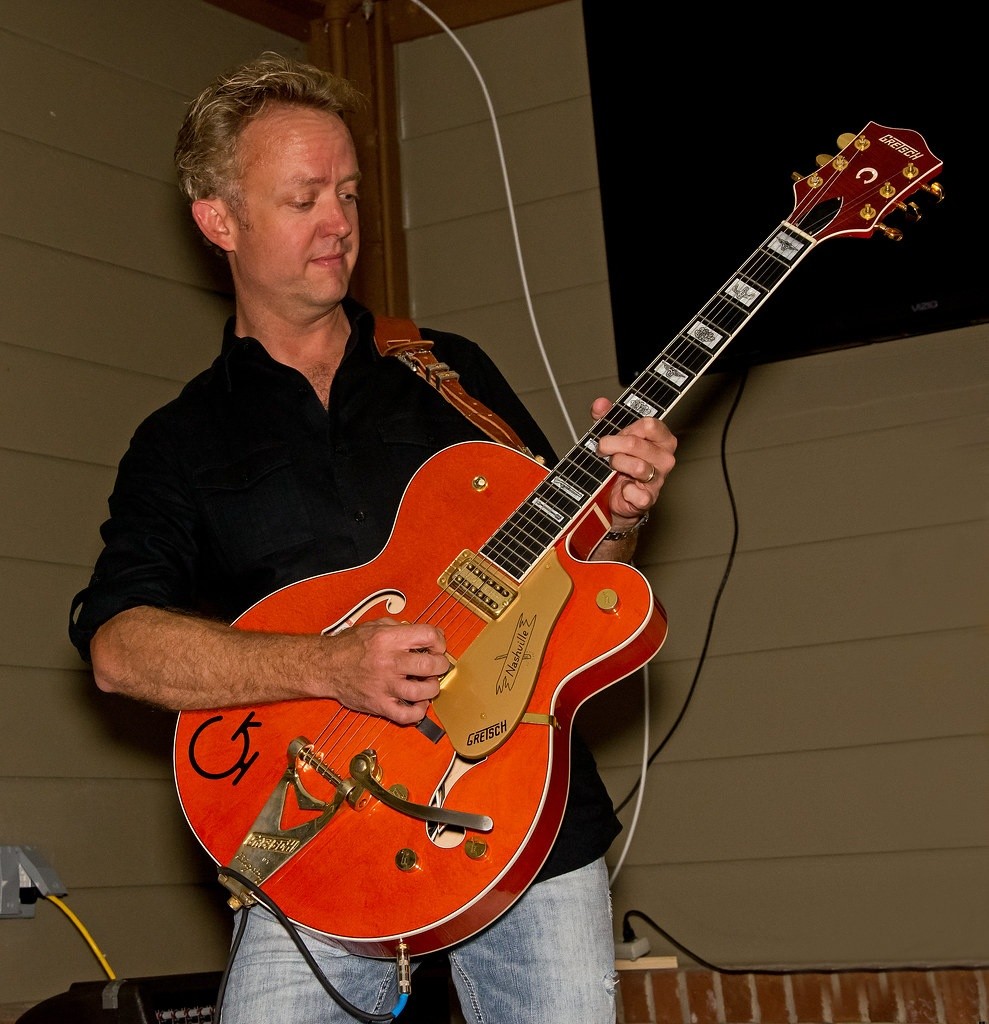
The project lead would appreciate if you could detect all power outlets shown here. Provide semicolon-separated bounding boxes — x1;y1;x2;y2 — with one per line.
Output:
0;845;69;919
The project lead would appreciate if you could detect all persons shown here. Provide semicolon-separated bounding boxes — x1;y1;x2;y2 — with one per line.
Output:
65;47;681;1023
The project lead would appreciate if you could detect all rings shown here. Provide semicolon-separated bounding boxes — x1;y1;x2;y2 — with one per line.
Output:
638;464;656;483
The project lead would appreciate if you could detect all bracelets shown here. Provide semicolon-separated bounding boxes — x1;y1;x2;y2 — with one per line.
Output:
603;508;650;542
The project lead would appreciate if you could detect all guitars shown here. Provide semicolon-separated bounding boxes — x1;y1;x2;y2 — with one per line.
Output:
165;117;948;961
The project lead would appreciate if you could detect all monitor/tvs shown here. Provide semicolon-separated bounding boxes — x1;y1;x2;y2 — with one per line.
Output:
580;0;989;387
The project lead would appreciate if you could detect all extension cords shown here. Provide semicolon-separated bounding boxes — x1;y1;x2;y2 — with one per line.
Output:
613;937;650;961
